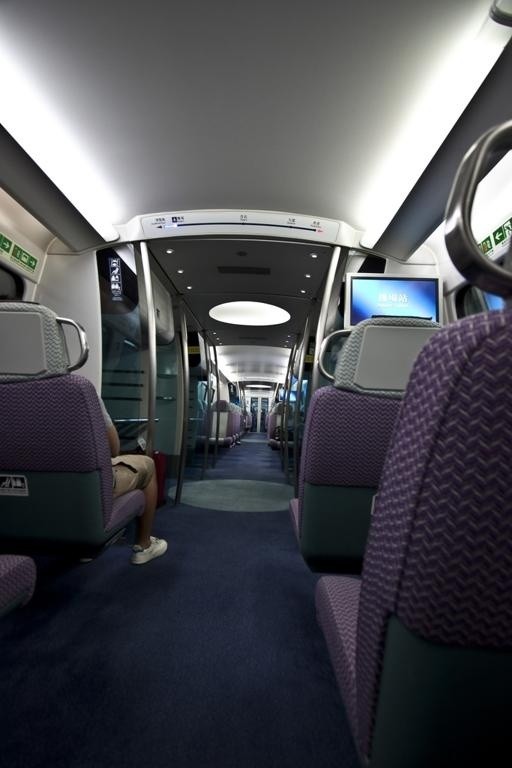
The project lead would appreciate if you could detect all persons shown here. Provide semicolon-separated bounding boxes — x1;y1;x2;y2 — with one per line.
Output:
98;392;167;565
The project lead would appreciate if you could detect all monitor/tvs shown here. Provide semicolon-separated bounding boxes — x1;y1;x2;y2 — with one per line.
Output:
343;273;443;331
279;387;297;403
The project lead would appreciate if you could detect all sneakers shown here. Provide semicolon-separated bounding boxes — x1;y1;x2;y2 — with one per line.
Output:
129;533;169;565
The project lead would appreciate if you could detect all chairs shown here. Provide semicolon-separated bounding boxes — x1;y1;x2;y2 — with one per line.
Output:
196;398;250;450
287;314;441;573
0;300;149;566
1;550;40;618
265;374;310;453
315;305;512;768
